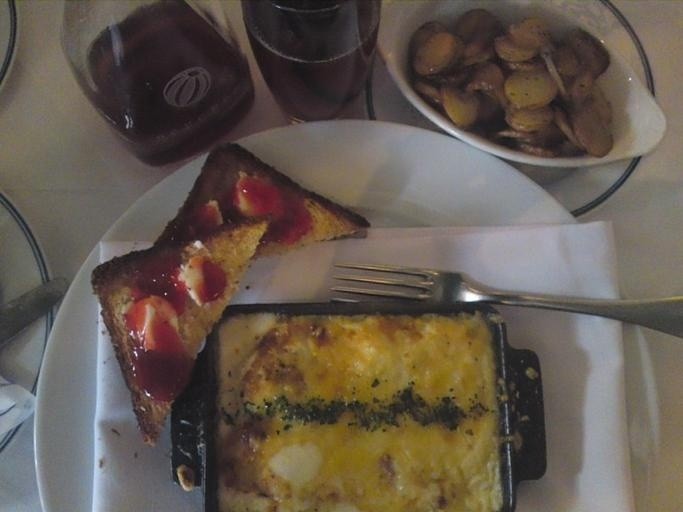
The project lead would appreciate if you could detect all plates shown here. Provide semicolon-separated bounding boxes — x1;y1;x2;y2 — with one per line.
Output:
0;2;18;85
380;6;667;167
1;193;50;451
31;119;660;505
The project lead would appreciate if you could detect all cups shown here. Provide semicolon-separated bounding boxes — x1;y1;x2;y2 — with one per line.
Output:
241;2;381;121
56;2;256;170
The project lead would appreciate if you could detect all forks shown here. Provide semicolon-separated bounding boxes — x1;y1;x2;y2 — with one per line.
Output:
328;259;683;338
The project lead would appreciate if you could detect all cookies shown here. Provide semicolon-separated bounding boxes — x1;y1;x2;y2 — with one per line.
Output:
414;8;611;157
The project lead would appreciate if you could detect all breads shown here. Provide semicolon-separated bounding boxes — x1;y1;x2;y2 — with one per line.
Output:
94;223;274;443
147;145;366;253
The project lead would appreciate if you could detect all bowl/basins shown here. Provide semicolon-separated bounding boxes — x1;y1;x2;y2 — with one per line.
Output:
365;2;657;222
